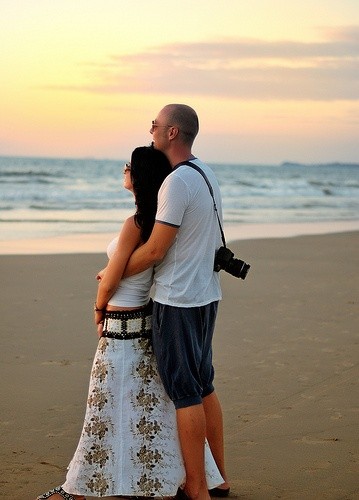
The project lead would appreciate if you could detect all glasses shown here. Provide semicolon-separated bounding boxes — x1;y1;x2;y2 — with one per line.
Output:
151;121;179;131
123;164;131;174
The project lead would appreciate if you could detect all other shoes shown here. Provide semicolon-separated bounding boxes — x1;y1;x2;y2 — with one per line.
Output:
173;488;230;500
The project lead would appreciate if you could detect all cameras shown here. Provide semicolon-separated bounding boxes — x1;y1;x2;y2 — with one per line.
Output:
213;246;250;280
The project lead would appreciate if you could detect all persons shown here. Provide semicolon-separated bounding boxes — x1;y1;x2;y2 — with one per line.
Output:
95;104;250;500
36;146;225;500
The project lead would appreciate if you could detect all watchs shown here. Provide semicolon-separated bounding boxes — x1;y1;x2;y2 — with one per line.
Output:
93;305;105;312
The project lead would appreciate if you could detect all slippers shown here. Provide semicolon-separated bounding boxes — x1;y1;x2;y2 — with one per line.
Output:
36;486;87;500
